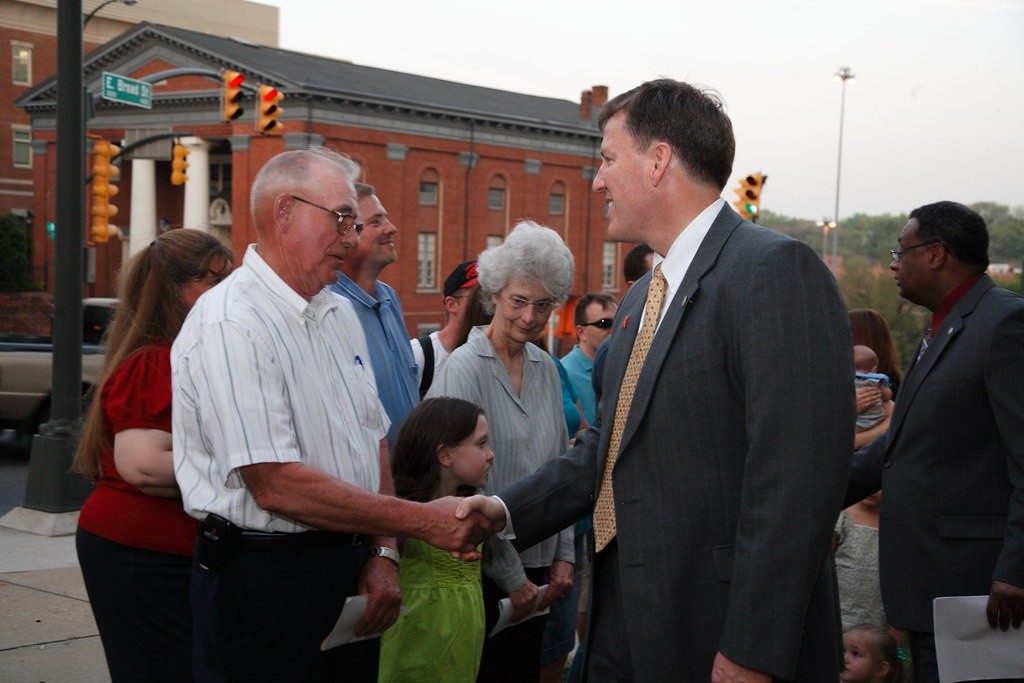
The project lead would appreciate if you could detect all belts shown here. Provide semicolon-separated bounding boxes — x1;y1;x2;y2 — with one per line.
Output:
233;527;373;553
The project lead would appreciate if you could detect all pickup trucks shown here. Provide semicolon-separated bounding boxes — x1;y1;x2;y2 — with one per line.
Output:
0;298;120;430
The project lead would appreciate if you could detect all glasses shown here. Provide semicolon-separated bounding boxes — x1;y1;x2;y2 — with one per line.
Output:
291;195;363;238
578;317;614;330
889;240;942;261
499;292;559;312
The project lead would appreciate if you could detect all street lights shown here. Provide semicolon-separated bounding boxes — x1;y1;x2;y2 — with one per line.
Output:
814;217;836;264
830;66;856;276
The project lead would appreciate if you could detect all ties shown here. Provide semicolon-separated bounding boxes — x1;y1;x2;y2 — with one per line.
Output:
910;322;934;378
591;264;668;553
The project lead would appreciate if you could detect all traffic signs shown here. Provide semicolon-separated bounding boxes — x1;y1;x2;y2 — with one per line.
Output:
102;72;152;110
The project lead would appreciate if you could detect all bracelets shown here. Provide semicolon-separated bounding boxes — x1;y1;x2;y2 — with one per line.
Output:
368;545;401;565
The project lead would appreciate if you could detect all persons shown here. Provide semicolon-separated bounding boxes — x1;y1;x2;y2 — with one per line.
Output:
446;78;857;683
75;147;906;683
842;200;1024;683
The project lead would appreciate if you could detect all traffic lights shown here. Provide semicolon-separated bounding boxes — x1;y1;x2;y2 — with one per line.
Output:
91;139;121;243
734;179;745;218
171;146;191;185
224;70;245;122
745;172;760;217
276;92;284;132
258;84;278;135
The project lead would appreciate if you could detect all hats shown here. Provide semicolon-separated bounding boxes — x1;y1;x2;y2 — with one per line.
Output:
443;259;481;298
